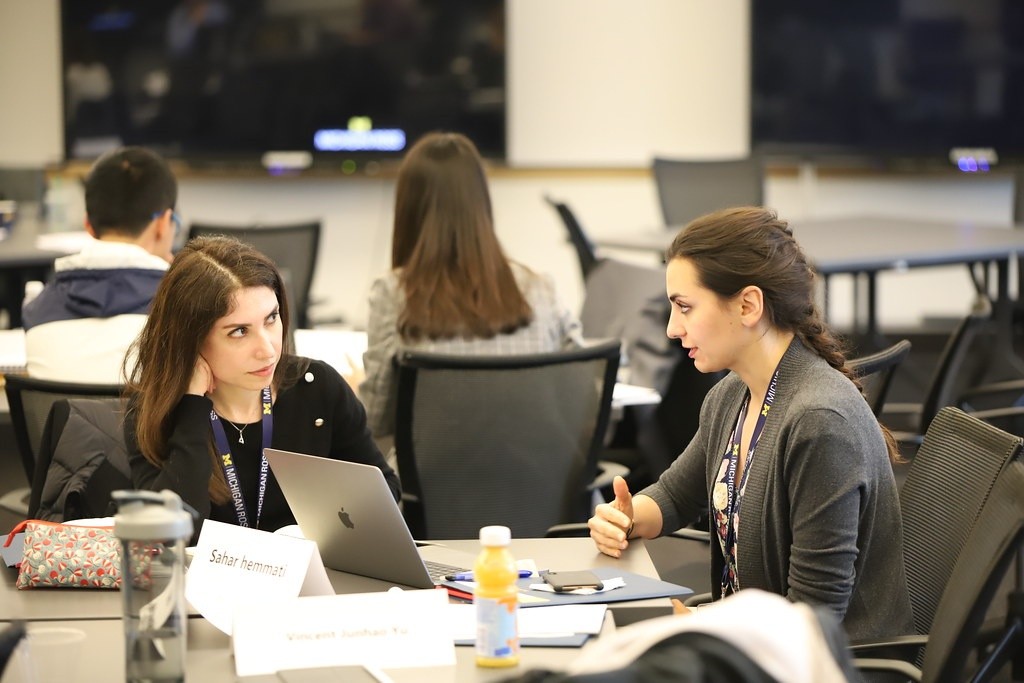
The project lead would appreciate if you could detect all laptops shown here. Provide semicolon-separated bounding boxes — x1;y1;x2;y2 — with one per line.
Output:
263;447;474;590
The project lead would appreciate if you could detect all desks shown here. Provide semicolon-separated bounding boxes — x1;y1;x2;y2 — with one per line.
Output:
584;209;1023;347
2;535;675;683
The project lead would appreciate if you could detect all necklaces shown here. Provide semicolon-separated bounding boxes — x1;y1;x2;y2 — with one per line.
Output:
212;402;262;444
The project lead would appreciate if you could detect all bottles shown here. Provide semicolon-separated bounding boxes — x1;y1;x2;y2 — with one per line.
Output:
469;524;521;668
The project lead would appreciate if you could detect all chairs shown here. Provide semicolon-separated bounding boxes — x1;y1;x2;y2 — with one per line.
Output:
546;517;715;608
183;220;325;328
651;155;766;328
830;406;1024;683
0;373;146;521
392;337;623;539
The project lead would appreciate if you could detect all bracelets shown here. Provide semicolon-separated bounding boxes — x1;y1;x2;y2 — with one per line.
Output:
627;522;634;539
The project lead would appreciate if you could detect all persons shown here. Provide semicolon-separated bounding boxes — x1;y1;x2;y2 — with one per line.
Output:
350;132;585;512
124;235;404;547
586;206;917;683
22;146;184;525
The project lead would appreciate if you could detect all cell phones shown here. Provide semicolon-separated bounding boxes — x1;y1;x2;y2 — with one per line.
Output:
542;570;604;592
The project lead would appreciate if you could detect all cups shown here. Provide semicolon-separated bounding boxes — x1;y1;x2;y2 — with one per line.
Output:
110;489;192;683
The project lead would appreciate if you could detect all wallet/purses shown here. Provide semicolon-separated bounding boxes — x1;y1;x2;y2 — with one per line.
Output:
5;519;152;590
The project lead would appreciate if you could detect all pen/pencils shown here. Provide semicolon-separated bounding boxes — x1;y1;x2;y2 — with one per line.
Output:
445;570;532;581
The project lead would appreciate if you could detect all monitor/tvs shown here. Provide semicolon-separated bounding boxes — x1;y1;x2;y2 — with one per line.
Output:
59;0;508;168
748;0;1023;171
